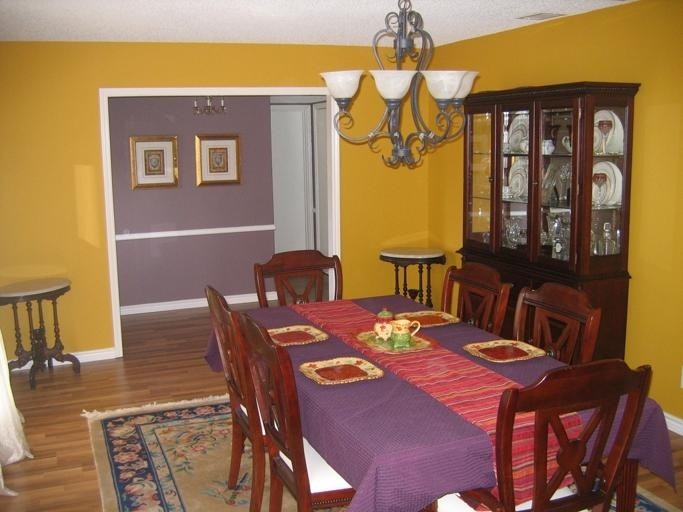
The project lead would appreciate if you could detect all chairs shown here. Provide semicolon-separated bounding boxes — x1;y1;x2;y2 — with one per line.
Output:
253;250;342;308
436;358;652;512
236;313;354;512
441;265;511;336
205;284;288;512
511;282;601;365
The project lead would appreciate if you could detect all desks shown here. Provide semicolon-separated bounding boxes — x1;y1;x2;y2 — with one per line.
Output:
1;276;80;391
378;247;445;307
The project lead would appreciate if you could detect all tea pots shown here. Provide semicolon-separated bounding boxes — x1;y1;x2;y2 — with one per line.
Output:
541;138;555;155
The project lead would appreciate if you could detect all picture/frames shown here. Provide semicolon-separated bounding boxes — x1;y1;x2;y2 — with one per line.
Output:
127;133;182;193
193;132;243;189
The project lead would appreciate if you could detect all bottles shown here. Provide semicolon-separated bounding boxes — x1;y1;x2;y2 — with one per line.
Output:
597;222;614;257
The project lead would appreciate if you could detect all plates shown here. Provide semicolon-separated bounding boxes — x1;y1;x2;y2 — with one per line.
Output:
393;310;461;328
592;161;624;206
265;324;331;349
508;157;530;200
297;357;386;386
593;109;625;156
508;111;529;152
461;339;547;364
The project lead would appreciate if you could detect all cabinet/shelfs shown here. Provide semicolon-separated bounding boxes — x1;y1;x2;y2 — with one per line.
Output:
453;81;643;364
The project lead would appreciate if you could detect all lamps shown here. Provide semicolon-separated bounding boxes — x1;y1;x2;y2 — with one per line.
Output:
190;96;228;117
318;0;481;171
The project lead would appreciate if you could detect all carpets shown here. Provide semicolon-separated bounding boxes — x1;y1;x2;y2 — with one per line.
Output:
78;394;682;512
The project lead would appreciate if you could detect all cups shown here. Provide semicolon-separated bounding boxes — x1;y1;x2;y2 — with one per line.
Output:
502;216;521;249
390;318;421;350
